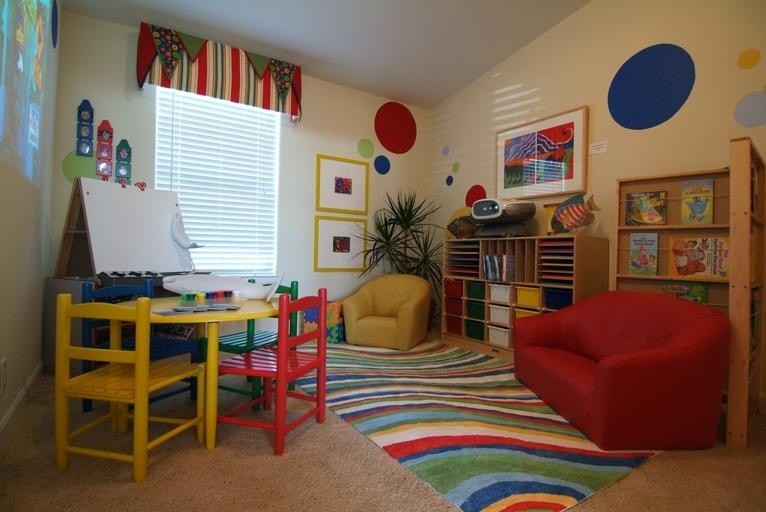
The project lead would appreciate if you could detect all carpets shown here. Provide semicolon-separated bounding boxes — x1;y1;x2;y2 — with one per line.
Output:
294;337;666;512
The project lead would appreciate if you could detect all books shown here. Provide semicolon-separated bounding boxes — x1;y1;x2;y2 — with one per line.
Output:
483;252;515;283
623;177;731;303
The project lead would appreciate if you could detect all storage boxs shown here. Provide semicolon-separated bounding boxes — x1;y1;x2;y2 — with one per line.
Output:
444;278;573;350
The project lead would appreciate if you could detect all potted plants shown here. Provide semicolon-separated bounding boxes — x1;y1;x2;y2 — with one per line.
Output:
348;190;453;332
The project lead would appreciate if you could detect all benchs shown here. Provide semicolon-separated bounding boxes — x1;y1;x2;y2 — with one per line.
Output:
513;289;732;451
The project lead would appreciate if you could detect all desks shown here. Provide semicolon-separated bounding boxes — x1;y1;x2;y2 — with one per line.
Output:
110;296;291;449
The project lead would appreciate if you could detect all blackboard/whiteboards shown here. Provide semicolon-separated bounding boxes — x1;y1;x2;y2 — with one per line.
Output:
80;177;197;278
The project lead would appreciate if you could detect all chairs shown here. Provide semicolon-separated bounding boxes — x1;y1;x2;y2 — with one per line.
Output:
197;280;299;410
340;274;432;351
81;276;203;430
204;288;329;456
52;293;205;483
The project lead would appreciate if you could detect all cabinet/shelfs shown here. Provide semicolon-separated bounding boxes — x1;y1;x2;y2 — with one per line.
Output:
441;235;611;361
43;271;210;376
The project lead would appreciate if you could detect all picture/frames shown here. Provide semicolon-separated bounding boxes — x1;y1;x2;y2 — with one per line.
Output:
494;104;590;201
312;216;368;273
313;153;369;215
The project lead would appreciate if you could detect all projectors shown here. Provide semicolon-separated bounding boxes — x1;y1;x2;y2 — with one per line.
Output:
471;198;536;223
